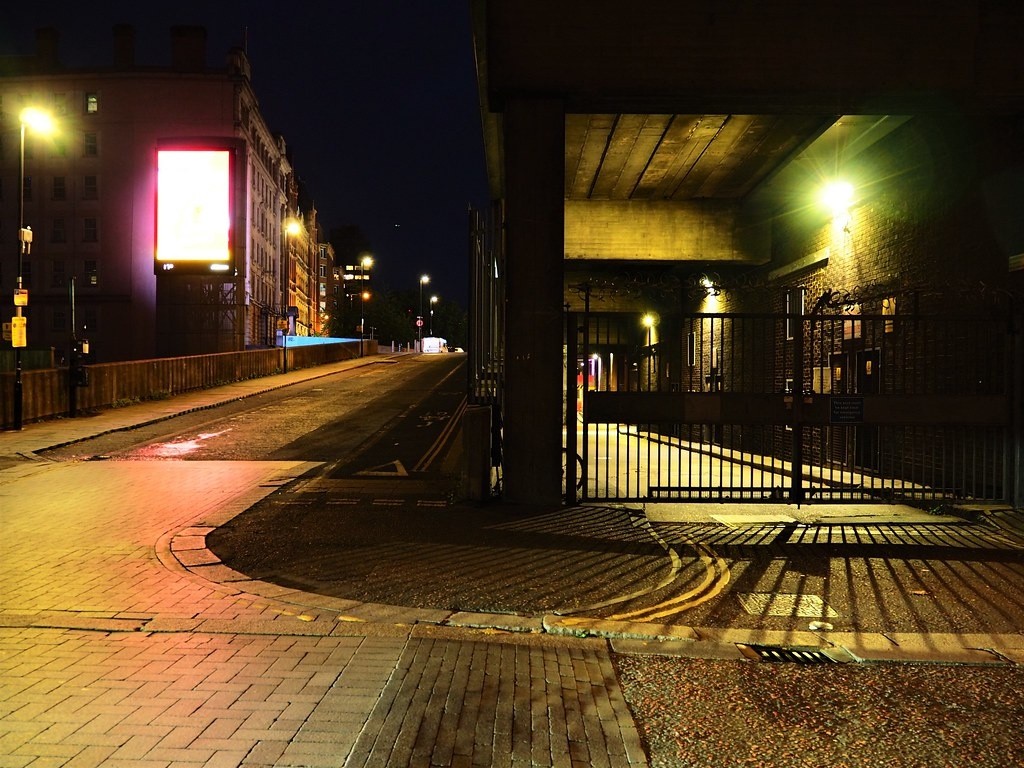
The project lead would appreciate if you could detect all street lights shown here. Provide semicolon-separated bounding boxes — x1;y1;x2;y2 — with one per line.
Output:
430;296;437;337
361;257;372;358
283;222;301;374
12;106;57;430
418;276;429;342
